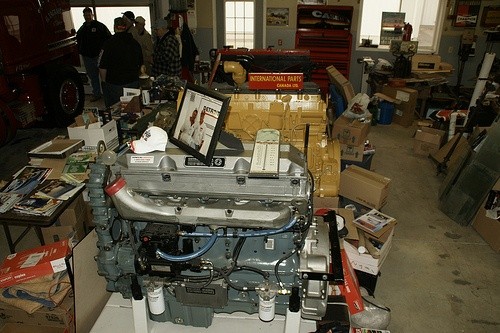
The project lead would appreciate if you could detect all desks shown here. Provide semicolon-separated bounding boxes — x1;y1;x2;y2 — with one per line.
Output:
370;71;448;119
0;101;168;252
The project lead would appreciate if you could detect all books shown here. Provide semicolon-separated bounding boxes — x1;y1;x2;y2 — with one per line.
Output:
62;152;91;174
0;167;85;216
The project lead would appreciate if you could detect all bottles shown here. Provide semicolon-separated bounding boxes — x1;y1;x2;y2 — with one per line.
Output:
150;77;156;102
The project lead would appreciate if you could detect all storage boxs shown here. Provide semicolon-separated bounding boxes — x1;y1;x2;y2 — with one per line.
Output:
312;41;471;297
0;93;144;333
472;208;500;254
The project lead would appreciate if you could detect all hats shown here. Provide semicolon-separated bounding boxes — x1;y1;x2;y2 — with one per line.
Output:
134;17;145;24
151;19;167;29
122;11;134;21
127;126;168;154
114;18;127;29
83;8;92;13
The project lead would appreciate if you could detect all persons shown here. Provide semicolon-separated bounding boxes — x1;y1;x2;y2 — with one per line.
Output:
98;17;144;108
164;13;200;82
121;11;135;32
151;18;182;80
178;109;206;152
76;7;112;102
131;16;153;87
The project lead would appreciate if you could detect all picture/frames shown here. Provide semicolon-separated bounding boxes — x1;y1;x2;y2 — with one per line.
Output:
166;80;230;166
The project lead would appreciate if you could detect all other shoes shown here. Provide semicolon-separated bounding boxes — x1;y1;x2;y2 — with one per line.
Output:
90;96;101;102
